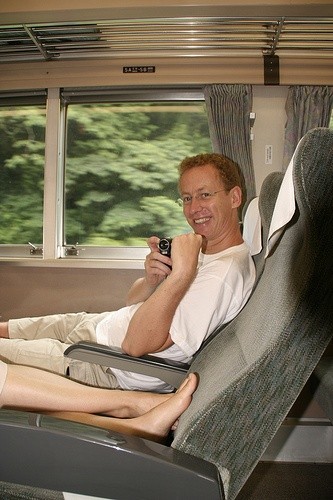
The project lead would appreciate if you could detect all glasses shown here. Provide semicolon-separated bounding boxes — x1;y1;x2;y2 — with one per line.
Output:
175;186;235;207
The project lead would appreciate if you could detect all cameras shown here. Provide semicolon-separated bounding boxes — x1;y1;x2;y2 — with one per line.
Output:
158;238;173;256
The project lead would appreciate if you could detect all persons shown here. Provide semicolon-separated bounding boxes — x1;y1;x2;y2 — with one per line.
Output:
0;152;258;391
0;361;199;445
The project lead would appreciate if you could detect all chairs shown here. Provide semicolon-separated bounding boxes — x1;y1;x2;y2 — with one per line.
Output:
0;128;333;500
